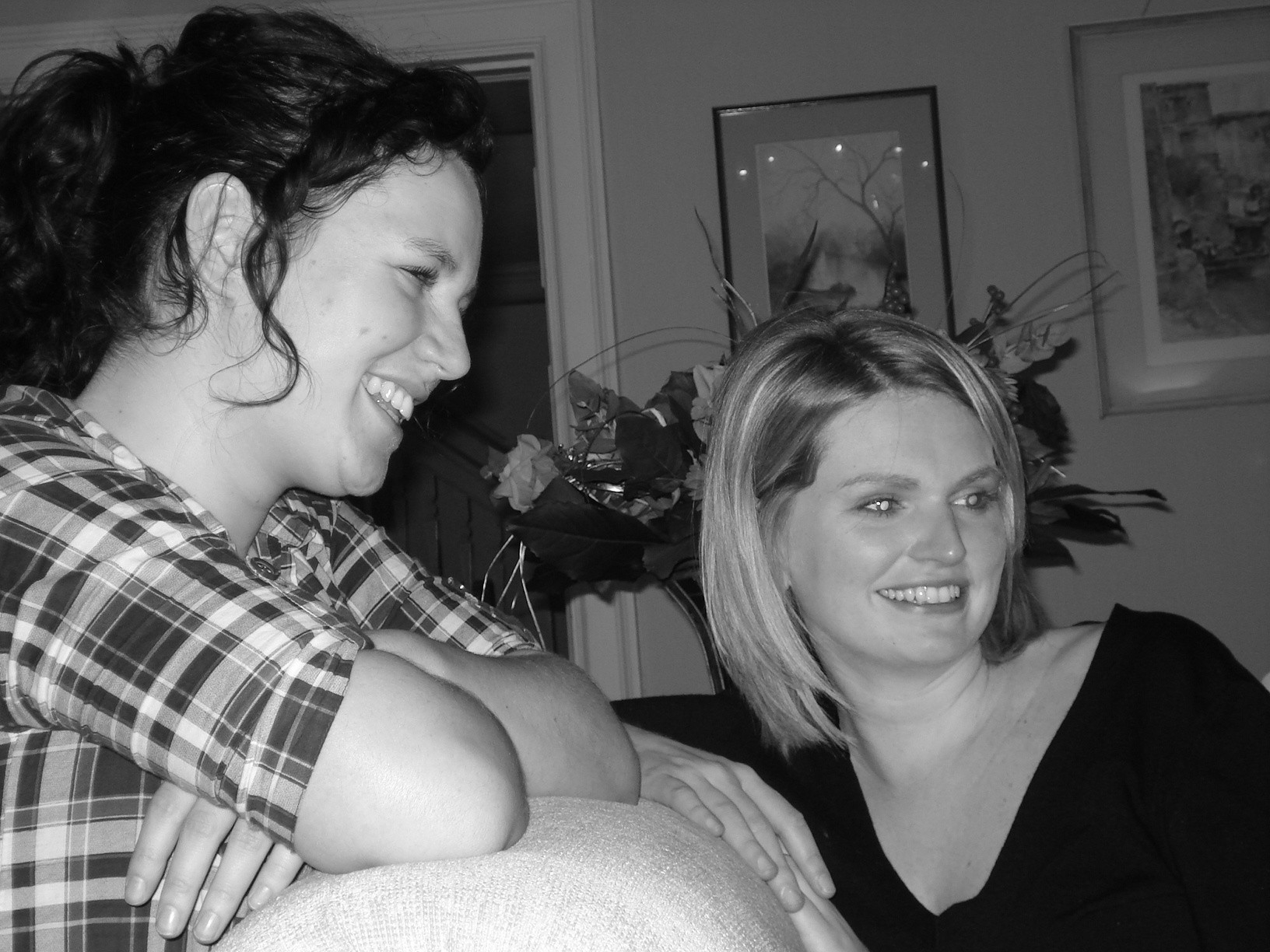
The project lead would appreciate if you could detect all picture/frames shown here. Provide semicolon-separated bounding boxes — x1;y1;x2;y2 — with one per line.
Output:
712;85;956;358
1068;5;1270;420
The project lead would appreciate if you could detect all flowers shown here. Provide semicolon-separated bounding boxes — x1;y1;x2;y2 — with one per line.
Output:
481;205;1168;583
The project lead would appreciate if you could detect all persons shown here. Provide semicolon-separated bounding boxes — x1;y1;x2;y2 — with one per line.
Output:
611;305;1270;952
0;5;641;952
